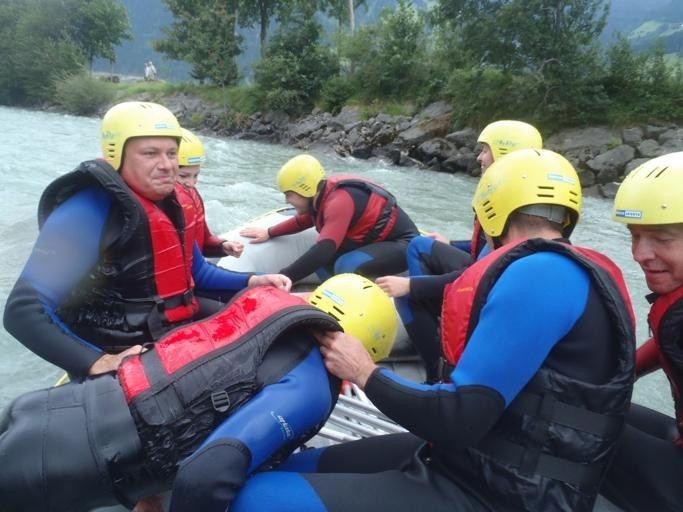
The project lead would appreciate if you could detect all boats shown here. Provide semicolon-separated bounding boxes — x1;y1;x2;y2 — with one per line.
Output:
93;209;626;511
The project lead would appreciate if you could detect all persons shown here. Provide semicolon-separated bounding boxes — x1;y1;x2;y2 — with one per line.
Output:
144;63;149;79
148;61;156;81
0;272;400;508
374;120;543;385
2;102;294;383
176;127;244;317
600;152;683;509
230;147;636;511
240;153;422;283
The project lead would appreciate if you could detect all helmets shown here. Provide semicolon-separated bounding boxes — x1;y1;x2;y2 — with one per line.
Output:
612;151;683;225
472;148;582;237
307;273;399;363
101;101;207;171
276;154;326;198
477;120;543;162
